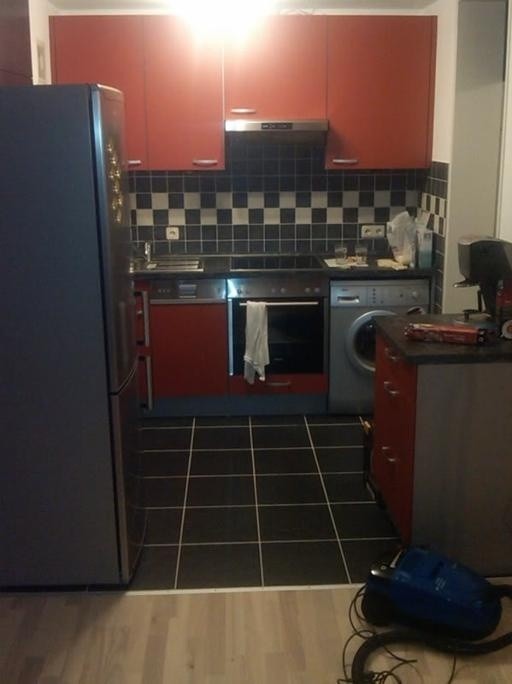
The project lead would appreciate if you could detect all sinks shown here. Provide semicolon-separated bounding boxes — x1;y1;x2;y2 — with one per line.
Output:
143;261;205;274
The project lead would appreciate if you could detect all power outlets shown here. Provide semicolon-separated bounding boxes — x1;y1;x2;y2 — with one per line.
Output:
361;225;385;238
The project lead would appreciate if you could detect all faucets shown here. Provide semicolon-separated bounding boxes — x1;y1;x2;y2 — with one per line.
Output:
143;240;151;262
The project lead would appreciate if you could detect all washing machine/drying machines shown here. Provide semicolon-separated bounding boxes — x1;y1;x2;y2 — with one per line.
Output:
328;279;430;416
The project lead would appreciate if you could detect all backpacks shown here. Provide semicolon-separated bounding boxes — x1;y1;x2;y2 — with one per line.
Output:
362;546;499;634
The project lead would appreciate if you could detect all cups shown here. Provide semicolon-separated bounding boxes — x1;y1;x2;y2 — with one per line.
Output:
354;243;368;264
334;243;348;265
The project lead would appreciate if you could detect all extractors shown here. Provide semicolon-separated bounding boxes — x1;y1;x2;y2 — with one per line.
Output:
225;120;328;147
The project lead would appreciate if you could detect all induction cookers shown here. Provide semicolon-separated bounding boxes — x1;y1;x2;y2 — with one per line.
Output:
230;254;328;273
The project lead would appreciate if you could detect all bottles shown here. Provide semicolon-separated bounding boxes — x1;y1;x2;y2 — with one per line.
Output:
495;267;512;333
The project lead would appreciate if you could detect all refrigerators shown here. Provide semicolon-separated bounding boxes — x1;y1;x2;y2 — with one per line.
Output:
1;84;153;588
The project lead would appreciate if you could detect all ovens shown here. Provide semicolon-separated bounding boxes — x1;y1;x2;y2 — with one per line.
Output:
227;297;331;376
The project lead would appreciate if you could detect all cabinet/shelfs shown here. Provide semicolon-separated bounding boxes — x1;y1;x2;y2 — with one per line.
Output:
51;15;150;173
326;15;435;171
222;12;326;122
129;253;331;419
141;13;225;173
368;314;512;578
